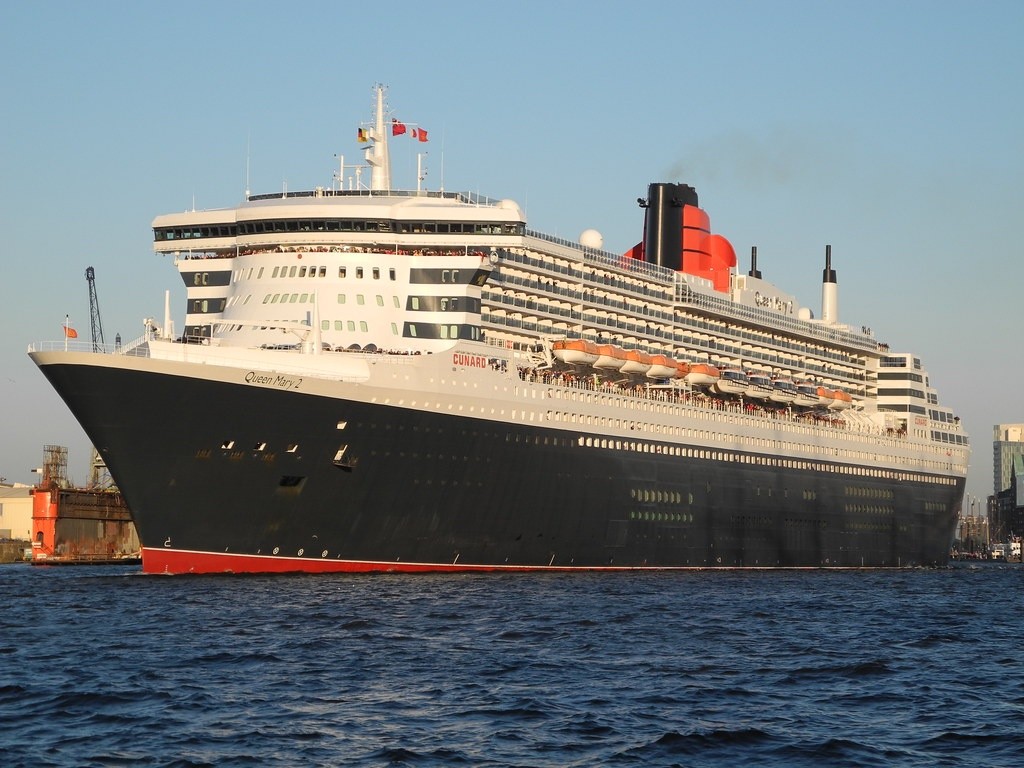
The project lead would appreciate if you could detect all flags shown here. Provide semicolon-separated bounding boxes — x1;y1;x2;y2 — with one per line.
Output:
412;128;430;143
64;326;77;339
357;128;369;143
391;117;407;137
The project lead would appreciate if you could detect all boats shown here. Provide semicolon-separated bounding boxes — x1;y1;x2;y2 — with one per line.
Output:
814;387;834;409
791;383;820;407
620;349;653;376
674;363;691;379
645;352;676;379
738;372;773;400
985;529;1022;564
829;392;853;412
768;378;798;404
592;342;627;371
709;367;749;396
553;337;599;369
686;362;719;387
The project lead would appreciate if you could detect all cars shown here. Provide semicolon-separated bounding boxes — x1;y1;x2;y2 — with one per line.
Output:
24;550;33;557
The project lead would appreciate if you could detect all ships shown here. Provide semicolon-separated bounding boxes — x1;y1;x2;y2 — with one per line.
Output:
27;79;973;572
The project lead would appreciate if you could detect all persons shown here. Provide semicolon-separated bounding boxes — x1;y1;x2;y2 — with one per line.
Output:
156;222;520;260
518;366;846;430
262;343;432;356
